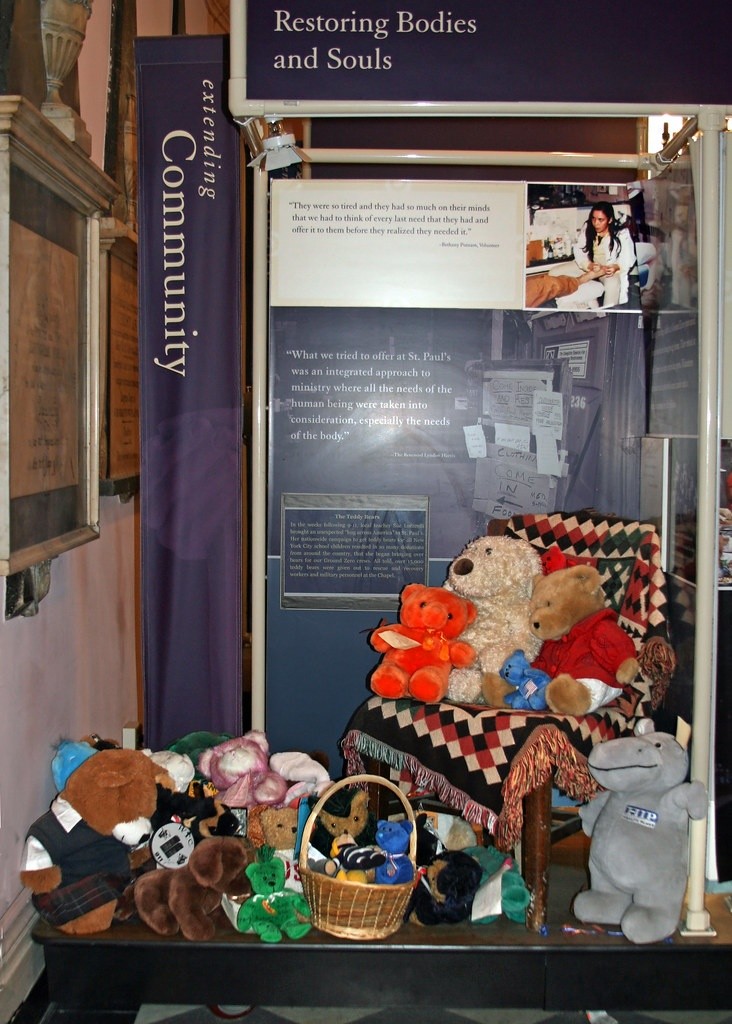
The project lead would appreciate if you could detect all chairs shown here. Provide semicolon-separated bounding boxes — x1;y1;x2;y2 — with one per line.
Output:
340;510;677;933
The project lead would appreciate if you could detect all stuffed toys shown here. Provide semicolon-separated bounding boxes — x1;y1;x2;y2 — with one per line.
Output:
26;730;531;942
115;837;252;938
375;818;413;884
483;564;638;716
573;718;708;946
237;845;311;942
261;807;304;895
461;846;530;925
19;748;175;936
325;833;385;874
369;582;476;703
499;649;551;710
541;546;576;576
443;535;543;703
406;851;481;925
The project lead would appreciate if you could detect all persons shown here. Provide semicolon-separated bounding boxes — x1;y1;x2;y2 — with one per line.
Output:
526;268;609;308
550;202;635;311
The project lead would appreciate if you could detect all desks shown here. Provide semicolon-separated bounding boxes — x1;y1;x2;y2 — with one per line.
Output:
33;847;732;1024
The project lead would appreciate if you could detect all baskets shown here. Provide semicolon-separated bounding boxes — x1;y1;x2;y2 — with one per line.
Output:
297;775;418;940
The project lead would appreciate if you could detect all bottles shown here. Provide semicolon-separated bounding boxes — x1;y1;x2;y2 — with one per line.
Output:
548;244;553;258
558;248;563;259
